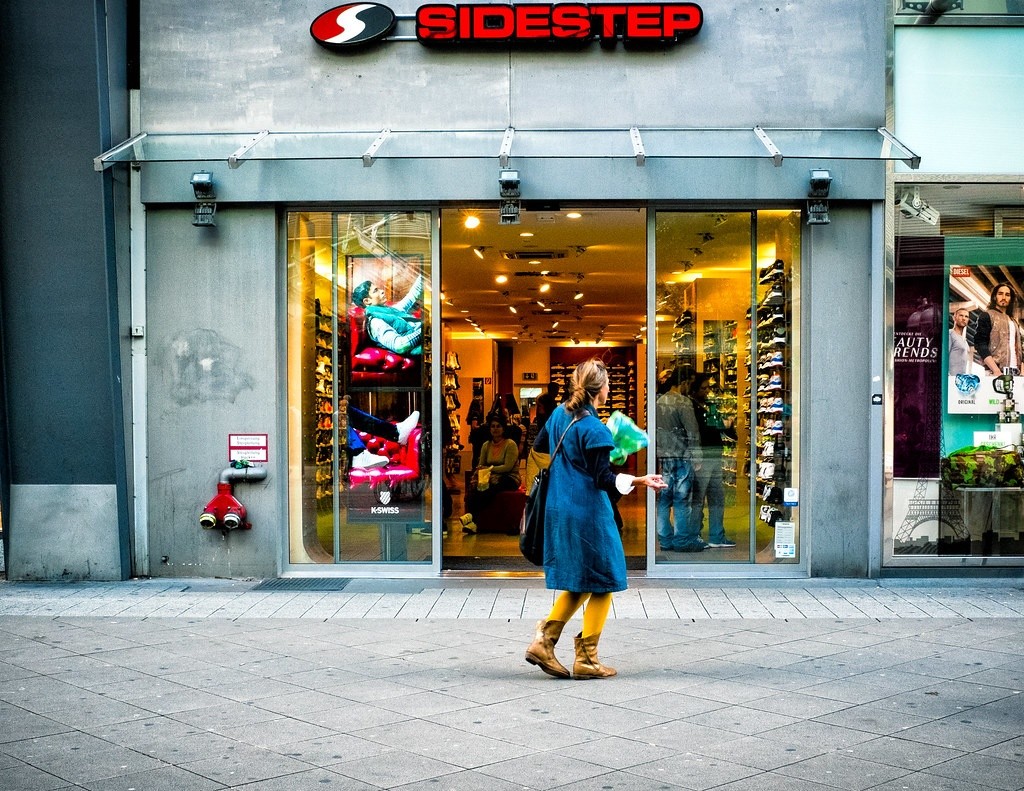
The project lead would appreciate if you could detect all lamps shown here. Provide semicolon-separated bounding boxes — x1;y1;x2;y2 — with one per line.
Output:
702;233;714;246
465;316;473;322
537;298;548;308
595;337;603;345
574;247;587;258
693;248;704;258
573;290;584;300
470;321;480;327
473;246;487;259
684;261;694;272
550;320;559;329
510;305;520;313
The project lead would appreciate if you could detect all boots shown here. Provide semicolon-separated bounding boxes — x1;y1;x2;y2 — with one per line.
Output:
526;620;570;678
573;631;617;680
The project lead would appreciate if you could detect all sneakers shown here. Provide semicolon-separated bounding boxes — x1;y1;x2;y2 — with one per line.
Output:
551;362;635;425
643;259;788;529
420;523;448;535
315;298;464;514
708;537;736;548
459;513;477;534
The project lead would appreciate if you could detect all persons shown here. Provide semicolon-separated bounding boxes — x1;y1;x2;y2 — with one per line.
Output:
547;382;571;404
459;412;521;535
974;284;1024;377
525;361;668;680
467;389;484;471
352;274;423;358
535;392;557;433
346;404;420;470
949;308;970;376
686;373;736;549
656;365;704;553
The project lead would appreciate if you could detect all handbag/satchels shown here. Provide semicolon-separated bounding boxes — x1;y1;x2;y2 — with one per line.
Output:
520;468;550;566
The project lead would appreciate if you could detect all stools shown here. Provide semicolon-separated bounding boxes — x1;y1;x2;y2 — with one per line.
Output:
475;482;529;536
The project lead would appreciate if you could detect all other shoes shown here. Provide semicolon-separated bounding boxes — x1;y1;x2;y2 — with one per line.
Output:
660;541;704;552
